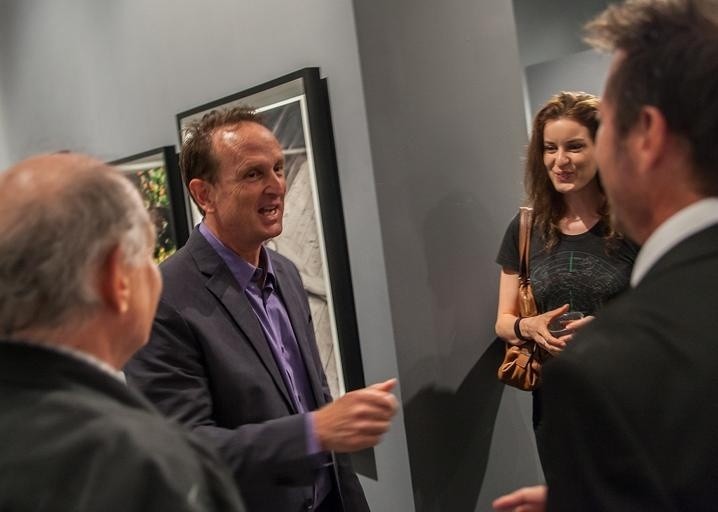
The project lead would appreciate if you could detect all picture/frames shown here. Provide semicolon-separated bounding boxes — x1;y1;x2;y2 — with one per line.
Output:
102;144;190;269
174;66;365;402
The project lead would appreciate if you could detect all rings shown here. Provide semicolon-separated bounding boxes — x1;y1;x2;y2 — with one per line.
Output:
542;341;547;346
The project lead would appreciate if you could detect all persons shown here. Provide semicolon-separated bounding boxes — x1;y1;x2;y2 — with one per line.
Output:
0;150;242;510
121;101;402;510
492;2;716;511
492;90;642;482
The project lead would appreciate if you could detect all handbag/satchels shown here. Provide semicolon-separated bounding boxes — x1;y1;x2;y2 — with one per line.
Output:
493;201;544;393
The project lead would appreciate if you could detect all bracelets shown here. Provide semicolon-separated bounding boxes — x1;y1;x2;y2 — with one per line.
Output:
513;316;531;343
